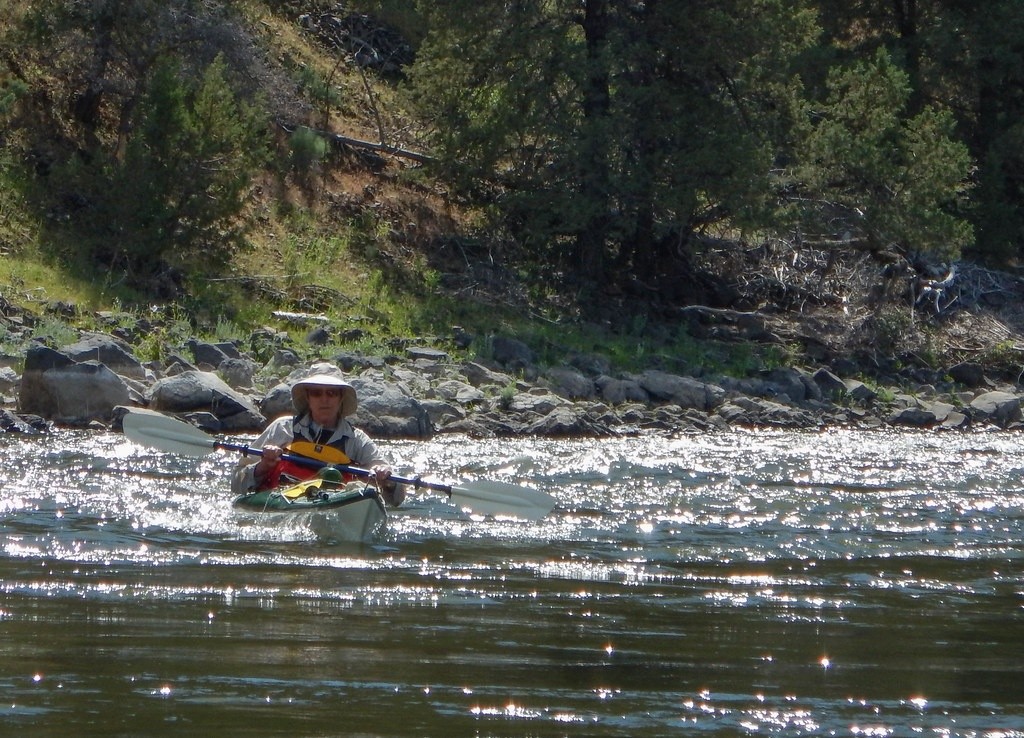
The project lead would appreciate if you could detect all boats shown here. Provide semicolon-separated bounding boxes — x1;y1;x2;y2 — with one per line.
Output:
231;475;390;548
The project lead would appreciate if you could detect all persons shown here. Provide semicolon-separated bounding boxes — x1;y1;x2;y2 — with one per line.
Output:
231;362;405;507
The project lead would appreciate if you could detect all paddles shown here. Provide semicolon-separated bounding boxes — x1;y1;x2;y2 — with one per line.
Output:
122;414;557;520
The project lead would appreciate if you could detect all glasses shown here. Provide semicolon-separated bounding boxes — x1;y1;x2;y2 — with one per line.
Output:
306;388;343;398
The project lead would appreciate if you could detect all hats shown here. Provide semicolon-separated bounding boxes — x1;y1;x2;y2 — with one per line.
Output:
290;363;357;419
